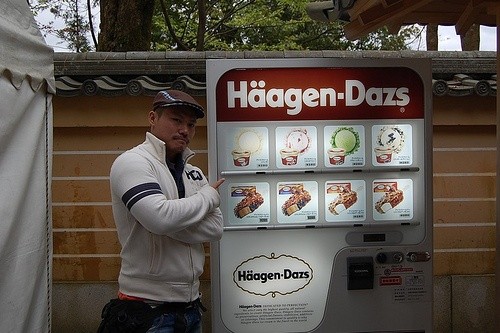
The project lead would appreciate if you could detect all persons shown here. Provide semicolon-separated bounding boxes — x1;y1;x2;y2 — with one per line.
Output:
93;88;227;333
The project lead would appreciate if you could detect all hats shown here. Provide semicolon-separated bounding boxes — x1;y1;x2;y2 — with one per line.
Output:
153;90;205;119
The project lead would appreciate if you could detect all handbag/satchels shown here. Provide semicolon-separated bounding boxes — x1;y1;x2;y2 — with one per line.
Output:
96;298;154;332
